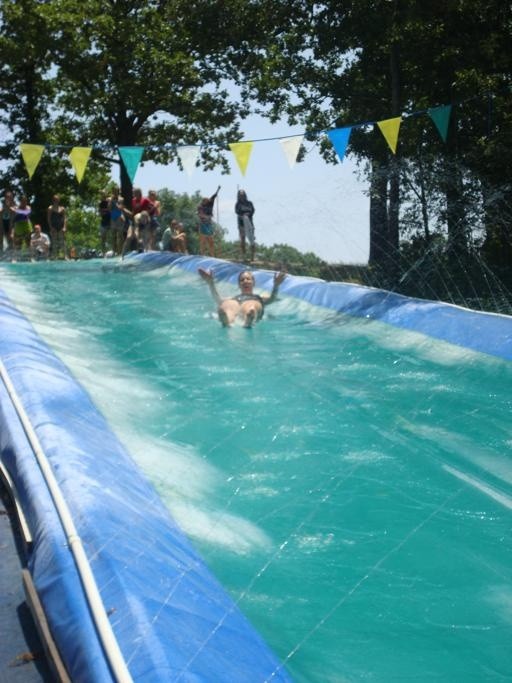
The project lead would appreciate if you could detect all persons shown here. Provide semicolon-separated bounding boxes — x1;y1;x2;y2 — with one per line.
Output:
196;266;292;333
0;184;284;266
